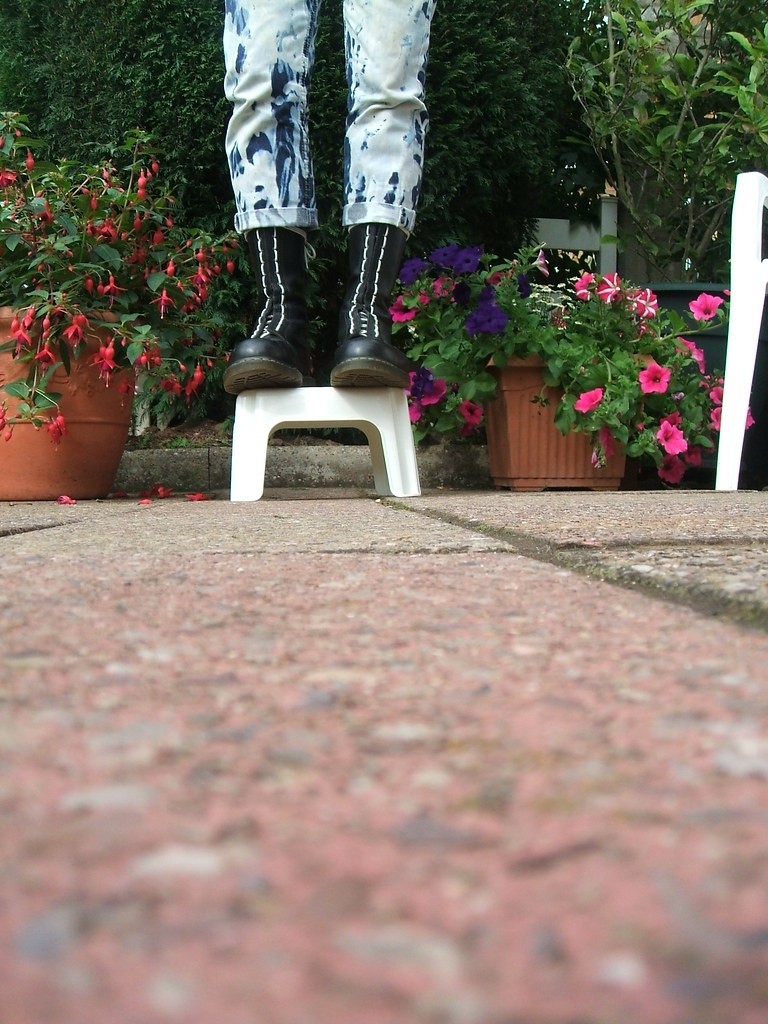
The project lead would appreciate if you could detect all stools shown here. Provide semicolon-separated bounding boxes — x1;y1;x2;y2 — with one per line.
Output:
230;386;422;501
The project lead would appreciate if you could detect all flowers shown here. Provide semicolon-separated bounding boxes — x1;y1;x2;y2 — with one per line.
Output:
388;244;757;484
1;111;239;447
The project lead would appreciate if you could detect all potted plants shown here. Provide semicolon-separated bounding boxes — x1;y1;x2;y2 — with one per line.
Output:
564;0;768;489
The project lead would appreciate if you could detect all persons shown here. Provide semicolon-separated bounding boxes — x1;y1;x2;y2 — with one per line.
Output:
222;0;438;395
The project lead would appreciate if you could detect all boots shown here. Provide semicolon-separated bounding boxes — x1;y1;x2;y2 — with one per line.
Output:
329;222;417;387
222;226;316;394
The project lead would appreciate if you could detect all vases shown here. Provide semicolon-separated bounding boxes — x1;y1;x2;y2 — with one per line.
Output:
0;305;136;500
475;355;628;492
